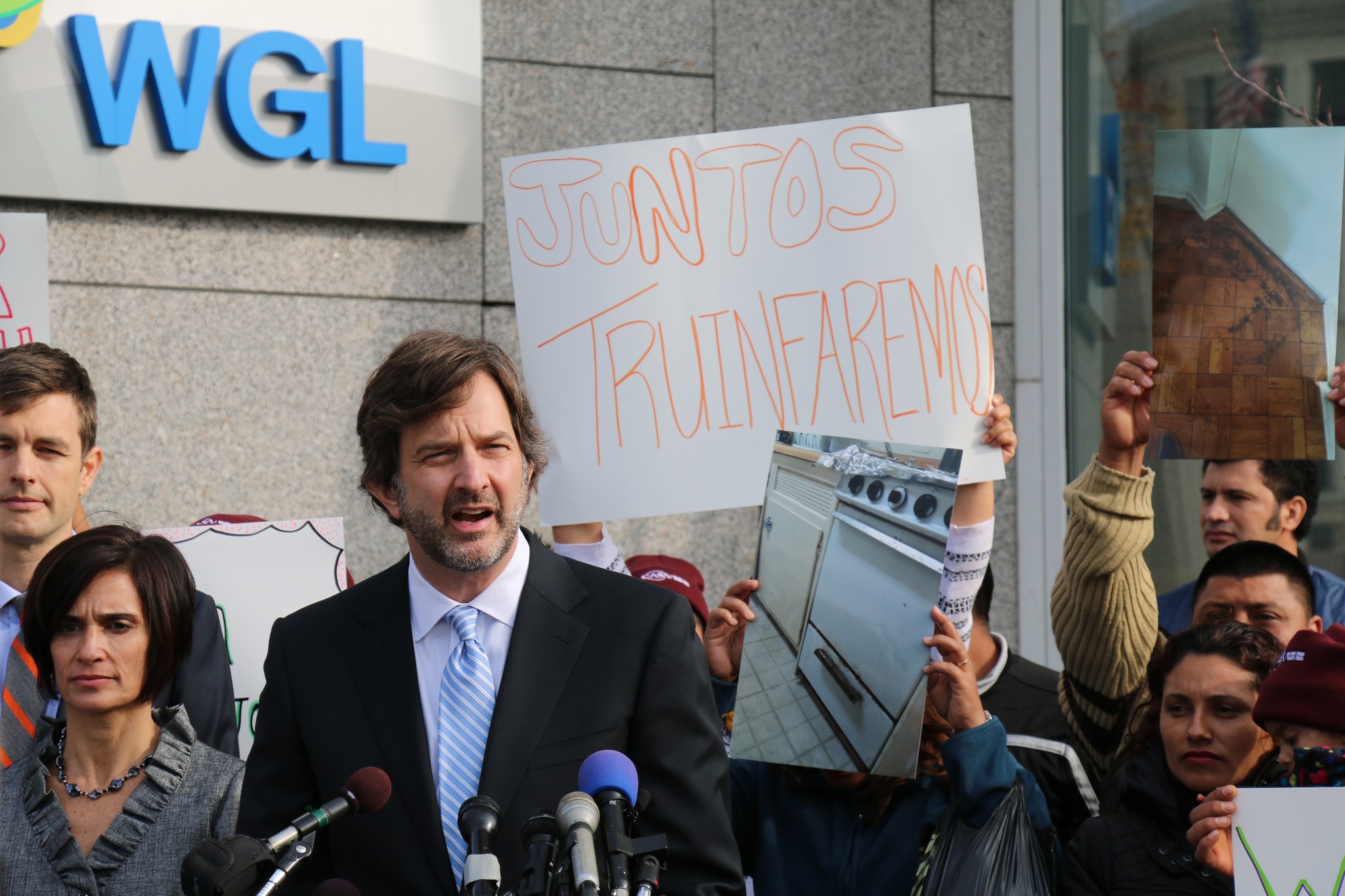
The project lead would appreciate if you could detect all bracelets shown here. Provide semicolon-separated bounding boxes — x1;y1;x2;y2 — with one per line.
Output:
983;707;994;725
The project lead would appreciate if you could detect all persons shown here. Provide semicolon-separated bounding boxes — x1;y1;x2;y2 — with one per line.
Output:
706;573;1054;896
1061;626;1286;895
227;330;750;896
1193;782;1234;878
1149;453;1342;638
960;561;1066;801
1262;628;1341;787
1;339;241;786
1046;348;1345;787
0;528;247;896
552;393;1015;669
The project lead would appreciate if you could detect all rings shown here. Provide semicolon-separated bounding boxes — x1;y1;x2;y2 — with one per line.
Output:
957;658;969;666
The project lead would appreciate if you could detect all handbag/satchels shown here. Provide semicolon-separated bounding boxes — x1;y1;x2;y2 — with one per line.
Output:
892;772;1053;896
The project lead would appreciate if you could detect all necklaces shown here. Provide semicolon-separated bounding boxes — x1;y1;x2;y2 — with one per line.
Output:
56;725;154;798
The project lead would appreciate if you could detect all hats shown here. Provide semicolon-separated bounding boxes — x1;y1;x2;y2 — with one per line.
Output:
1248;622;1345;737
623;556;709;627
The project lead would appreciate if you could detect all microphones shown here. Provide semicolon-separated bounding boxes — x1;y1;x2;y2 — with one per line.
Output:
267;765;392;856
522;807;557;896
556;788;604;896
579;749;638;895
459;794;502;896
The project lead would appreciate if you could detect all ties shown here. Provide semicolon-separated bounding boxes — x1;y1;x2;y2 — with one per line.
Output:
437;605;495;892
0;595;45;775
